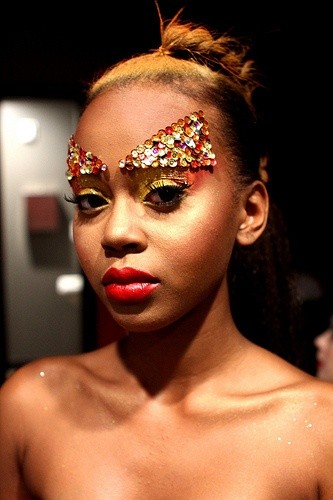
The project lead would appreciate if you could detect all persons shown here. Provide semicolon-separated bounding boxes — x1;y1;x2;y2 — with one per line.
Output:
312;323;333;386
0;0;332;500
